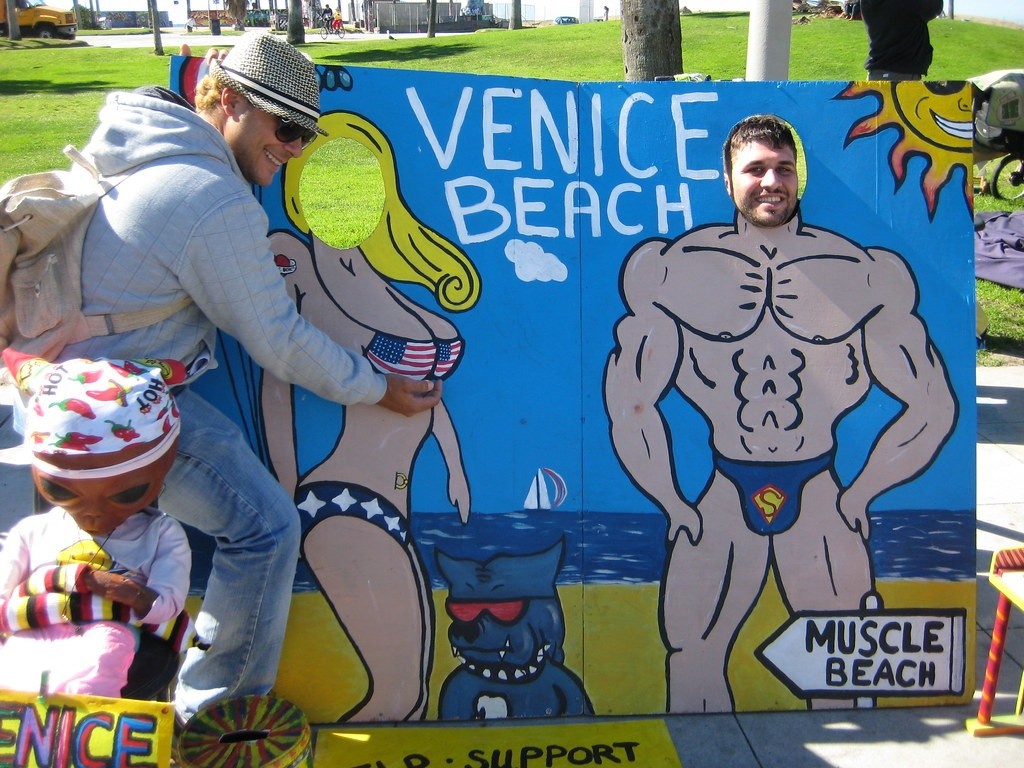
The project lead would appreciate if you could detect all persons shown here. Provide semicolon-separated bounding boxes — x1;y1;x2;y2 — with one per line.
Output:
185;15;197;32
844;0;860;21
726;117;799;227
13;31;443;732
861;0;943;80
1;347;190;699
321;4;342;34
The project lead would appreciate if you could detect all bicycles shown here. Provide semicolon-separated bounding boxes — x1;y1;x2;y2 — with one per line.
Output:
320;17;345;40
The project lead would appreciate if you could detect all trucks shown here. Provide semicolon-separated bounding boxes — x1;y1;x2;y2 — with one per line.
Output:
0;0;78;40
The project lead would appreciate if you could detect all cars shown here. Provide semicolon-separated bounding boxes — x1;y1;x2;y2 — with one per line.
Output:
553;16;579;25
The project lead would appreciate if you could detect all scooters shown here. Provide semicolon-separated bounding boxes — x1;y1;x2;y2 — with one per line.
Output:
974;101;1024;200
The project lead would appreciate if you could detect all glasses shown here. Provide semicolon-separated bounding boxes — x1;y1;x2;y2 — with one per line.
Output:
275;121;317;150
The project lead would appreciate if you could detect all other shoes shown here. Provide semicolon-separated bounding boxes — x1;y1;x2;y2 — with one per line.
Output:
148;676;184;760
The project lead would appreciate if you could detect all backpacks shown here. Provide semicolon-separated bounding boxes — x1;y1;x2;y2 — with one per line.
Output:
0;145;221;363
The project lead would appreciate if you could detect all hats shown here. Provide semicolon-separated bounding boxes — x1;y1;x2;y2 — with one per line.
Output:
209;30;329;138
